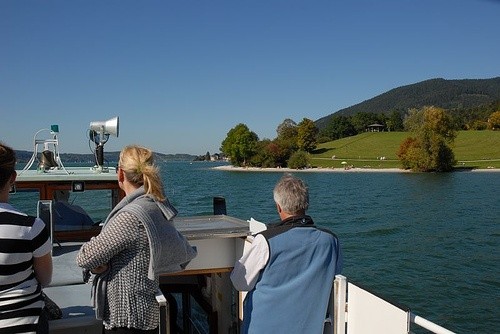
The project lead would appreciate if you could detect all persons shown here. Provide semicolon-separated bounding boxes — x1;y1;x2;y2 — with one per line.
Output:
0;144;54;334
229;173;344;334
76;144;197;334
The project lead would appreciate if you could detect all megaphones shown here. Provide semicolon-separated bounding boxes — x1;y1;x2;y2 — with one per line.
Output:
89;115;119;137
